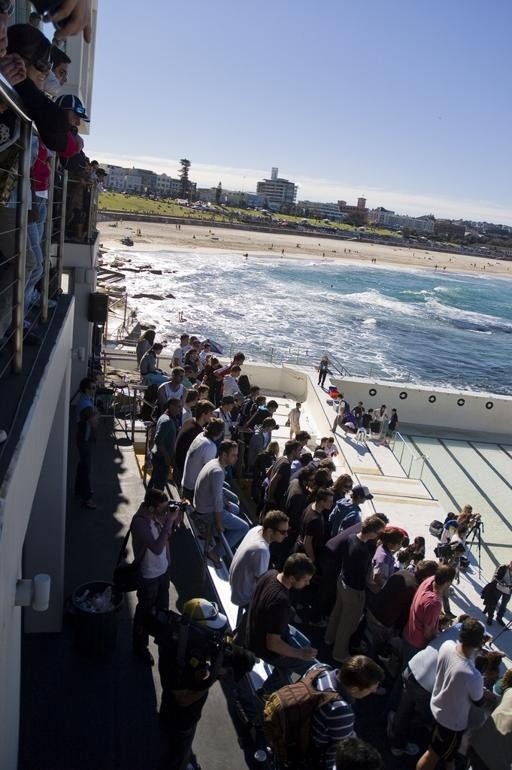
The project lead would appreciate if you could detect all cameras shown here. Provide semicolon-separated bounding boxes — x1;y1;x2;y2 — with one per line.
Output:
168;499;188;512
230;426;236;432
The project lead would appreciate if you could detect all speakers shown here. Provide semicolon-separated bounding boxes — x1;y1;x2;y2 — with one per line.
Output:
88;292;108;322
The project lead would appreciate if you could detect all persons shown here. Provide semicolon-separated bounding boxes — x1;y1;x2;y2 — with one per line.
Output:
1;0;108;342
70;329;512;770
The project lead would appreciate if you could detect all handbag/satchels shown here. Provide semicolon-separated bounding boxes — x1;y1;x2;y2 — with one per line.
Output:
293;536;327;577
113;558;138;592
251;471;266;506
289;625;311;648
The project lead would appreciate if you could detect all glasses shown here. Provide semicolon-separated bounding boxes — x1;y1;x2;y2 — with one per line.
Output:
197;602;219;620
34;43;59;73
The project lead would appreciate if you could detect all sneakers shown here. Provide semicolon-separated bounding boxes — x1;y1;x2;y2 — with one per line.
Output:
386;710;396;738
86;499;96;509
32;289;56;308
309;616;329;628
208;550;220;569
391;743;419;757
142;647;154;664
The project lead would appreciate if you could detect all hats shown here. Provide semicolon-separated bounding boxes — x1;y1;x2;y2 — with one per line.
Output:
352;485;373;499
223;396;238;403
183;598;227;629
56;94;88;119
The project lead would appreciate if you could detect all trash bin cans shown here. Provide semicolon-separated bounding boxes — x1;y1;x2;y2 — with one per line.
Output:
72;580;125;660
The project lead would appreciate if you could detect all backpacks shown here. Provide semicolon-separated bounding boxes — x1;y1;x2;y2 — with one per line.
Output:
263;667;334;761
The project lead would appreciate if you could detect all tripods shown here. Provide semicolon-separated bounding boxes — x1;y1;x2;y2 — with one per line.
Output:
462;522;485;580
